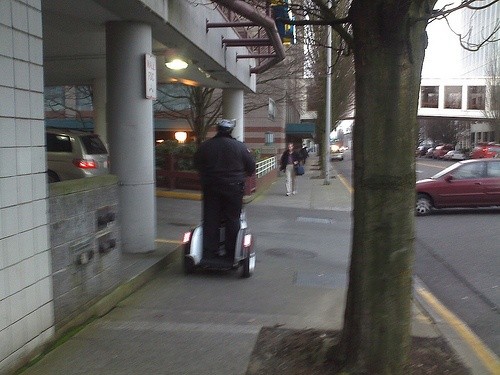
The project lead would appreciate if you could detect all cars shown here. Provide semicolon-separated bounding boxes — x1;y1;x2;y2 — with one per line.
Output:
469;145;500;160
417;144;449;160
330;149;344;161
443;150;466;161
415;158;500;217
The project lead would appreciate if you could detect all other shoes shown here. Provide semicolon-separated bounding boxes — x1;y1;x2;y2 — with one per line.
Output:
292;190;295;194
286;193;291;196
225;250;235;260
203;248;218;260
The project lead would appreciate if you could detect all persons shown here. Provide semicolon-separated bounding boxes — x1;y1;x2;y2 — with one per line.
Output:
279;142;302;196
437;142;455;161
301;148;309;166
472;141;485;159
485;142;496;158
193;119;255;264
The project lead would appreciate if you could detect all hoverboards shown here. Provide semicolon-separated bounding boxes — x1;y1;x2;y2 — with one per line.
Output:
181;171;256;278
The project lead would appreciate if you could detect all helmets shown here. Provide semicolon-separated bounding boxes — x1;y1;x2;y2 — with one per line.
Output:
216;119;234;134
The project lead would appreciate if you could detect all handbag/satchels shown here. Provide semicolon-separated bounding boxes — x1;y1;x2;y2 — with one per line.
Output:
295;160;305;176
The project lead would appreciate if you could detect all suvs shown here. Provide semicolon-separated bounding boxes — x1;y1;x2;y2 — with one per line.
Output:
45;126;109;186
